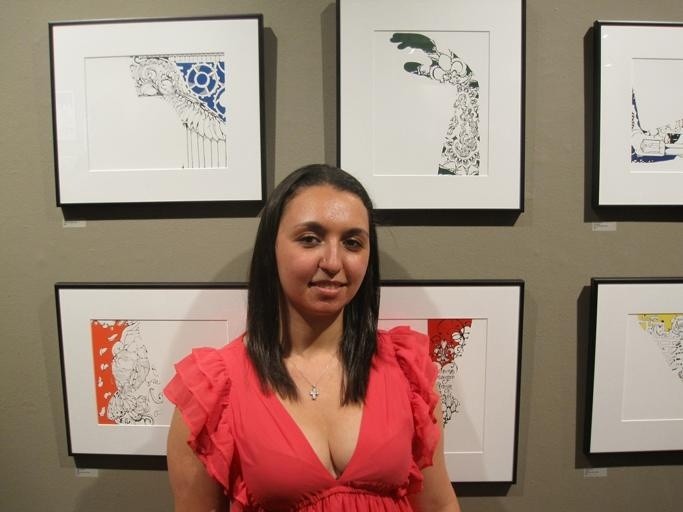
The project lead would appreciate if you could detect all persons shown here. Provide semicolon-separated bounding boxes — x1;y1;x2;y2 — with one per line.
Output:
160;163;461;512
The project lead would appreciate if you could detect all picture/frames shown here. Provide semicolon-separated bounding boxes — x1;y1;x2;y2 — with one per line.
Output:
377;278;526;484
584;277;683;456
591;21;683;208
53;279;248;458
47;12;267;208
334;1;527;215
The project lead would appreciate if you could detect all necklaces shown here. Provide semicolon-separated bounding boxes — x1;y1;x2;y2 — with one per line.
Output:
283;353;341;401
